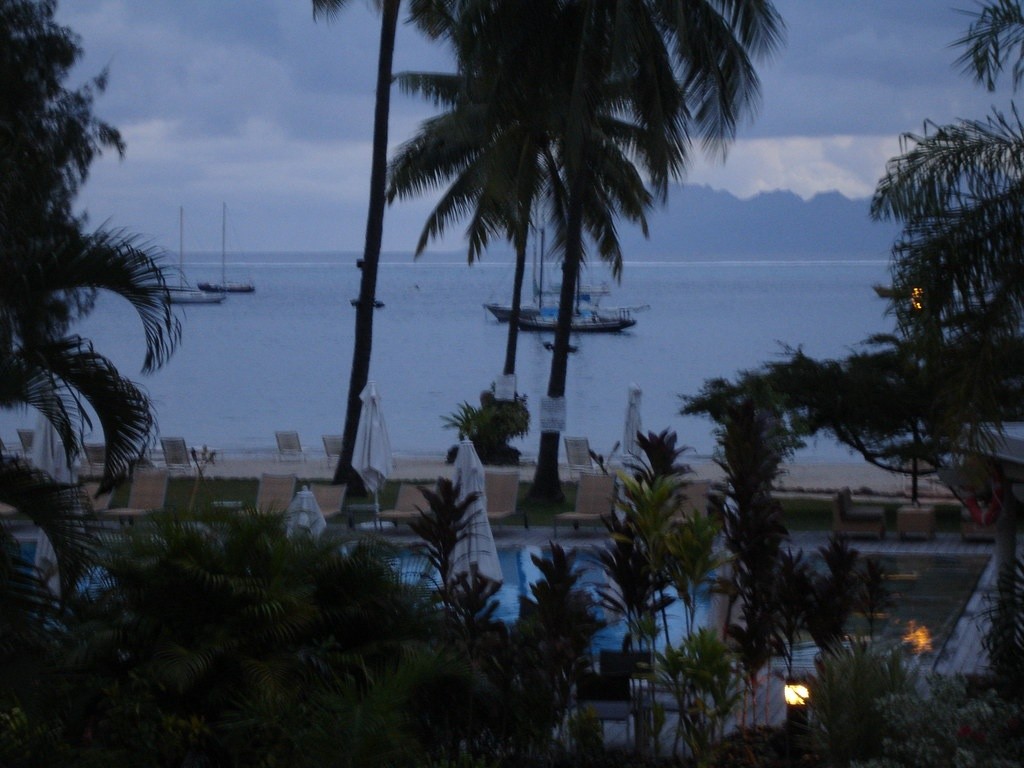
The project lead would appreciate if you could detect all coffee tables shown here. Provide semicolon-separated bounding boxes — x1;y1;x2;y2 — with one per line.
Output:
897;504;938;542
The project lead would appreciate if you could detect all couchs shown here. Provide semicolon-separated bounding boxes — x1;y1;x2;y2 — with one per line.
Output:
961;497;1005;542
831;486;886;540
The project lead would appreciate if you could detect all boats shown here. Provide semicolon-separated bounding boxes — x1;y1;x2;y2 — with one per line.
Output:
483;226;651;333
872;282;906;298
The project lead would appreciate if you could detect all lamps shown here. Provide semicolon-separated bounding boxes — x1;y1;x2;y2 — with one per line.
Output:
785;676;809;722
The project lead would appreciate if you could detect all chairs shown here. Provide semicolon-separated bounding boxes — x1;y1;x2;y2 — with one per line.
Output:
484;472;529;533
159;437;207;472
241;473;297;514
0;502;19;529
68;484;112;521
99;468;169;527
19;429;108;467
309;483;346;518
569;674;634;744
554;473;616;539
599;648;652;720
373;483;437;531
675;480;710;525
320;435;343;468
275;432;309;464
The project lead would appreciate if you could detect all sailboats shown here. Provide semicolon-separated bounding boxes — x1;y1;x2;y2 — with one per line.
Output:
151;205;226;303
195;201;255;293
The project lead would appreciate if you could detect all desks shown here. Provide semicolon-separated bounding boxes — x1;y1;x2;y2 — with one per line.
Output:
213;500;240;513
